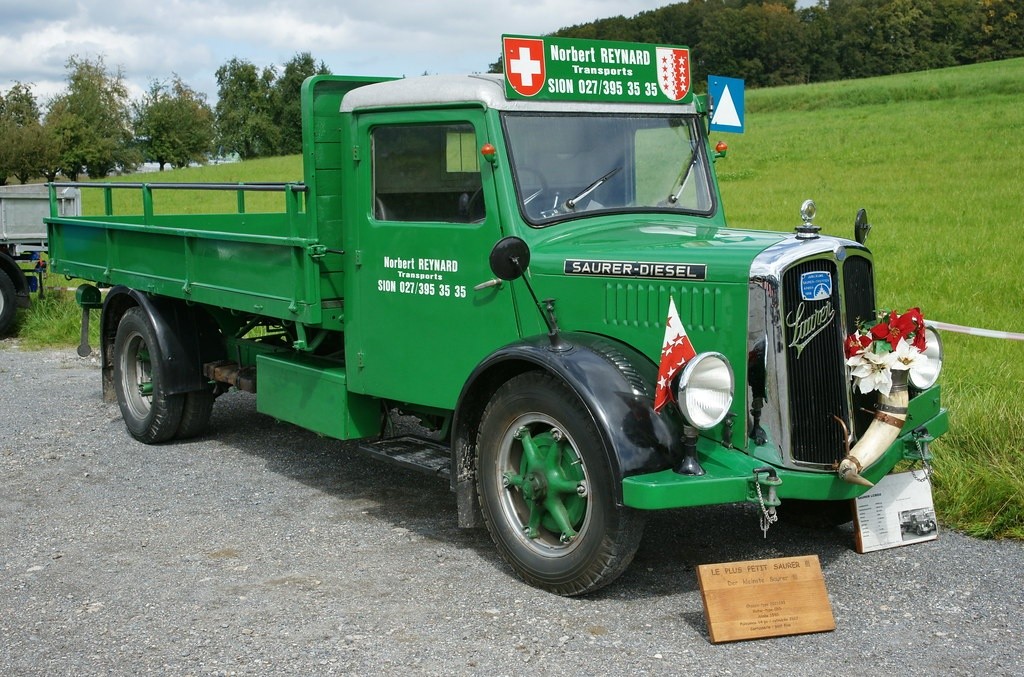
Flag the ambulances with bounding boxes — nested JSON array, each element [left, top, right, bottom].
[[44, 32, 949, 598]]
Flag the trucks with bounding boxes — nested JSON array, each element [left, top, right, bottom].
[[0, 181, 83, 339]]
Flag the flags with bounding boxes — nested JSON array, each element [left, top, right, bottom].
[[653, 299, 695, 413]]
